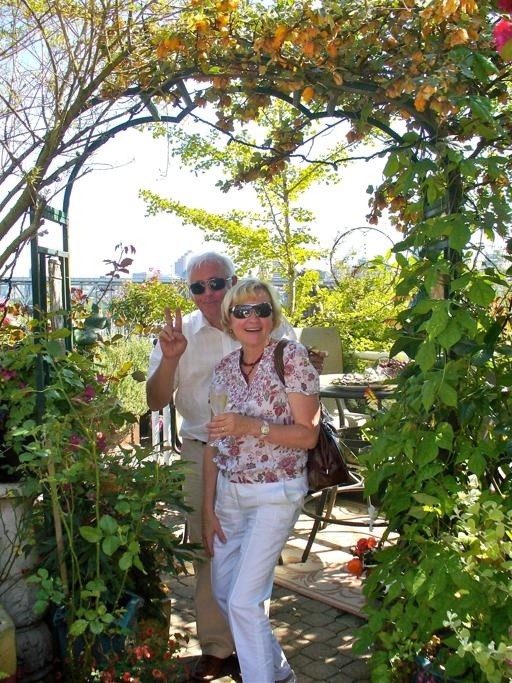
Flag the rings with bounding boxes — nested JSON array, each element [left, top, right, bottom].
[[221, 425, 225, 432]]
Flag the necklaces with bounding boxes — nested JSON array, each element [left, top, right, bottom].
[[239, 348, 263, 366], [239, 359, 254, 381]]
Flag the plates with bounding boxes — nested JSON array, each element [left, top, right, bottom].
[[332, 379, 388, 391]]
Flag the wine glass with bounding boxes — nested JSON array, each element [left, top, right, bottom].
[[207, 385, 230, 449]]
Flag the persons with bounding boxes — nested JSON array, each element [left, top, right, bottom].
[[144, 250, 329, 683], [201, 278, 324, 683]]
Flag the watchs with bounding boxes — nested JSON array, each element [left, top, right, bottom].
[[257, 417, 270, 440]]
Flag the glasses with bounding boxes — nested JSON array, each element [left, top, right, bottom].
[[229, 302, 274, 318], [188, 277, 232, 293]]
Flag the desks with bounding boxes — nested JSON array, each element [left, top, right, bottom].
[[300, 374, 402, 563]]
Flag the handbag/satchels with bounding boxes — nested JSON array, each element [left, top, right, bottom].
[[307, 416, 352, 495]]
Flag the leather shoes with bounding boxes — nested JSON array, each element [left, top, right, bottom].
[[191, 653, 224, 682]]
